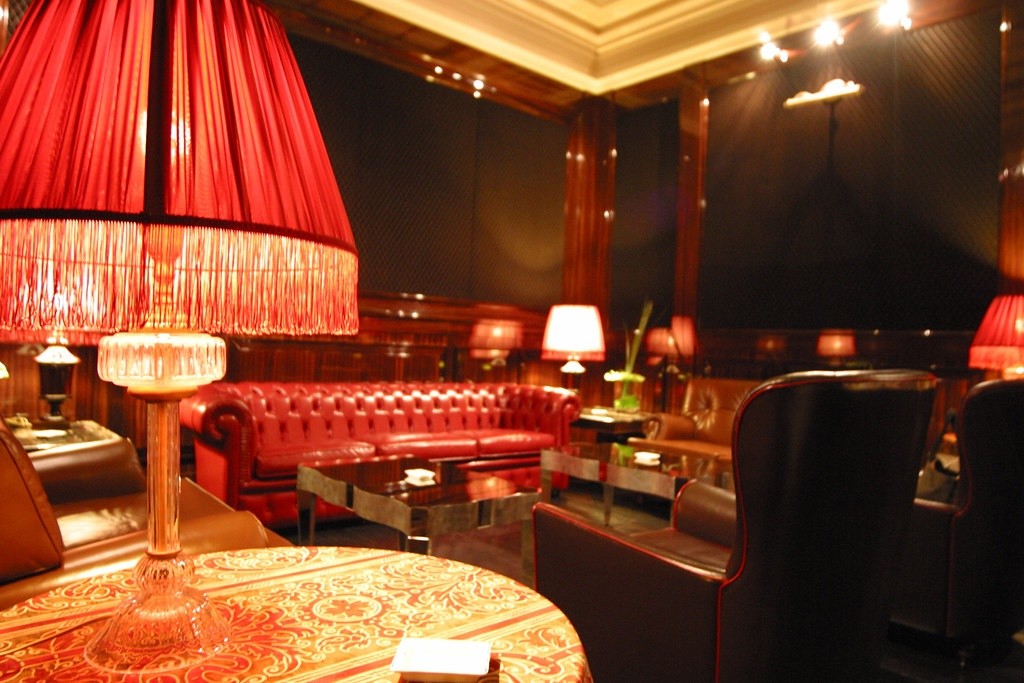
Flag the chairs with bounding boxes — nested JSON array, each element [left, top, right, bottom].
[[0, 421, 269, 610], [532, 369, 1024, 683]]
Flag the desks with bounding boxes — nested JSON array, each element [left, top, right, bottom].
[[297, 458, 543, 557], [4, 418, 121, 450], [0, 546, 593, 683]]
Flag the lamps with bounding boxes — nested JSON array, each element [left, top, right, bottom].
[[967, 294, 1024, 378], [0, 0, 360, 674], [540, 305, 605, 372], [33, 345, 80, 423]]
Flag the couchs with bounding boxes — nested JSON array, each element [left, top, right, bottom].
[[633, 379, 948, 492], [179, 382, 583, 527]]
